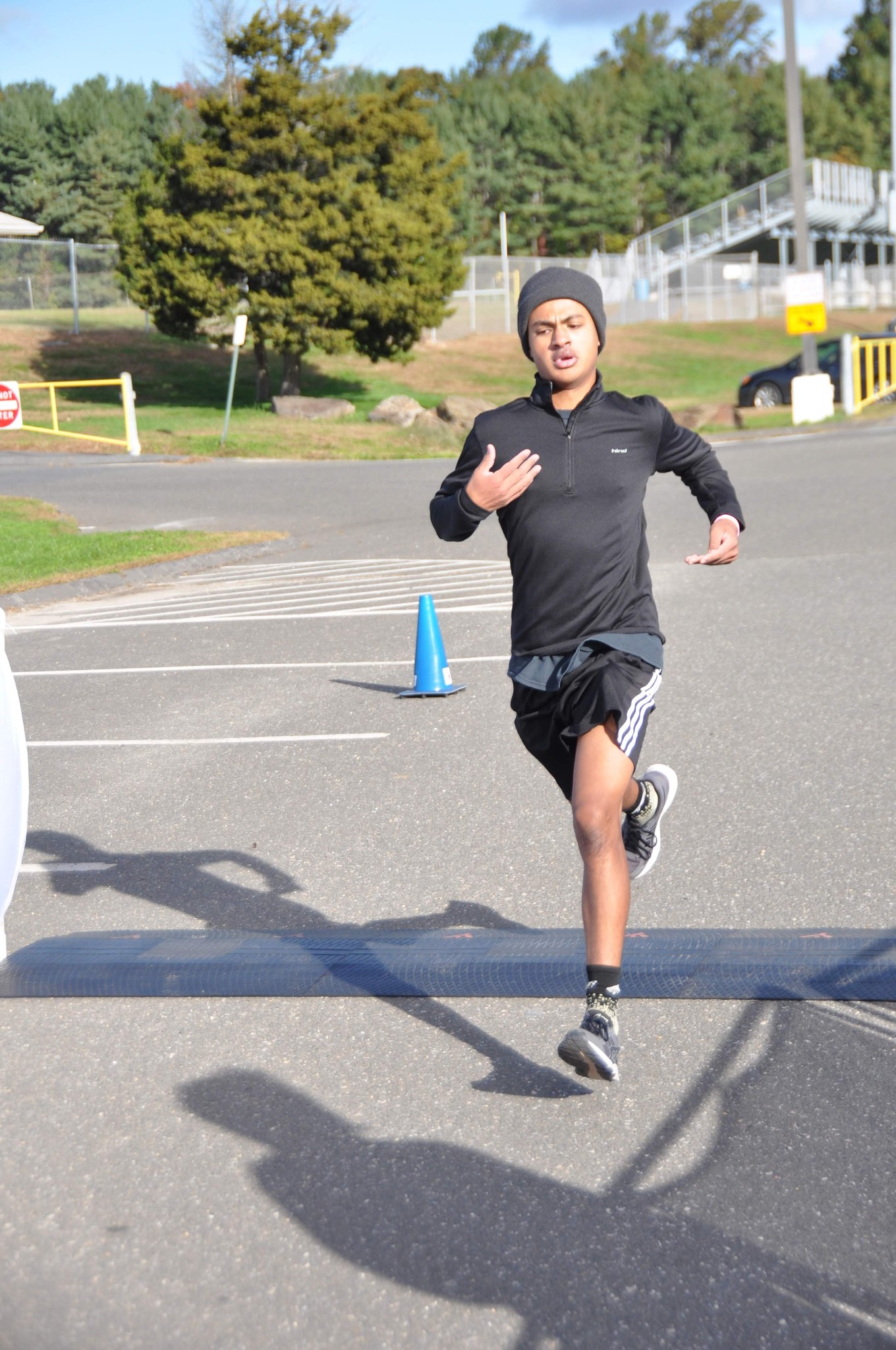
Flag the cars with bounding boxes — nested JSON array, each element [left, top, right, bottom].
[[738, 333, 895, 408]]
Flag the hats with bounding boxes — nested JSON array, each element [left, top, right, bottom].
[[518, 266, 606, 362]]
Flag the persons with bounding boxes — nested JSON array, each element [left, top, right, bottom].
[[427, 267, 744, 1083]]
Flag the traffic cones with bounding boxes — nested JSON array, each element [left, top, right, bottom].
[[395, 595, 465, 698]]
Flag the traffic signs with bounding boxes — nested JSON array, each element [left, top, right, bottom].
[[0, 372, 27, 432]]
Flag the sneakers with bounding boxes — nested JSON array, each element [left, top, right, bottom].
[[621, 763, 678, 879], [558, 1014, 620, 1081]]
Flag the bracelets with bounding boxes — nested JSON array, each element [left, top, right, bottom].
[[713, 514, 740, 537]]
